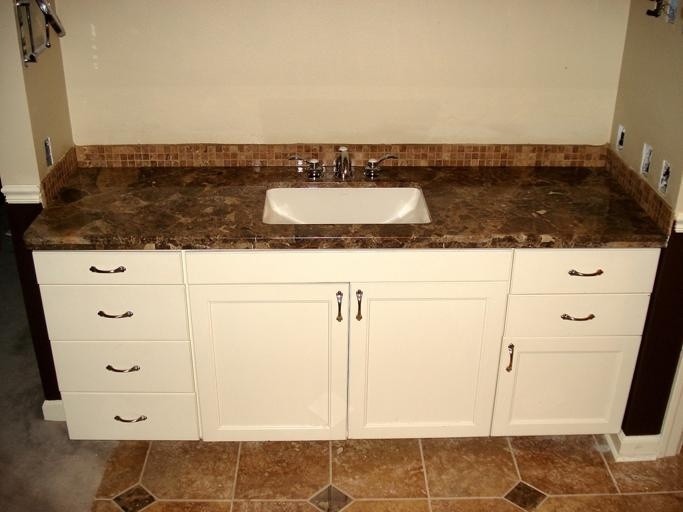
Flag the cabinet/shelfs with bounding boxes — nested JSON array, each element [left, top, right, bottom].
[[28, 250, 202, 439], [490, 249, 662, 440], [184, 250, 514, 440]]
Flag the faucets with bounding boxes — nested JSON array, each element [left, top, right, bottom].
[[336, 146, 353, 180]]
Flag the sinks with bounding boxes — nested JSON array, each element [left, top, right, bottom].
[[262, 181, 433, 225]]
